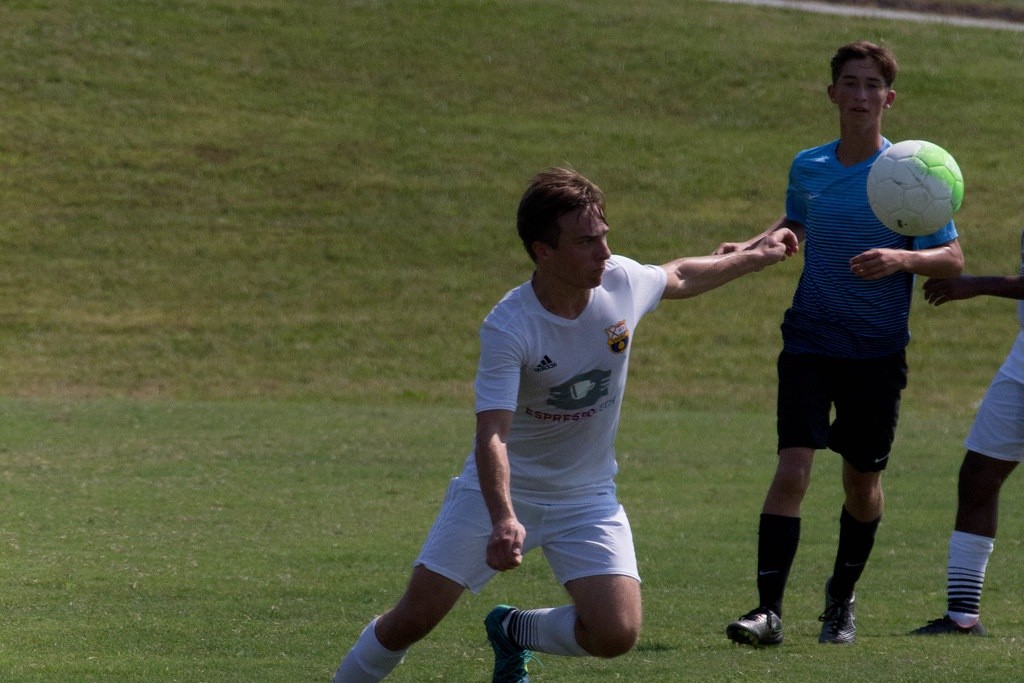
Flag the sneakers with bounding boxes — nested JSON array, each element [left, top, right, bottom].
[[483, 604, 545, 683], [817, 576, 855, 643], [909, 614, 985, 636], [726, 606, 784, 646]]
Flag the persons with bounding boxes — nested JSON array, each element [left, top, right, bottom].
[[713, 43, 965, 648], [910, 227, 1024, 637], [331, 167, 799, 683]]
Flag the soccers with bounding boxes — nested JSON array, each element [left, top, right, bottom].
[[865, 138, 968, 239]]
